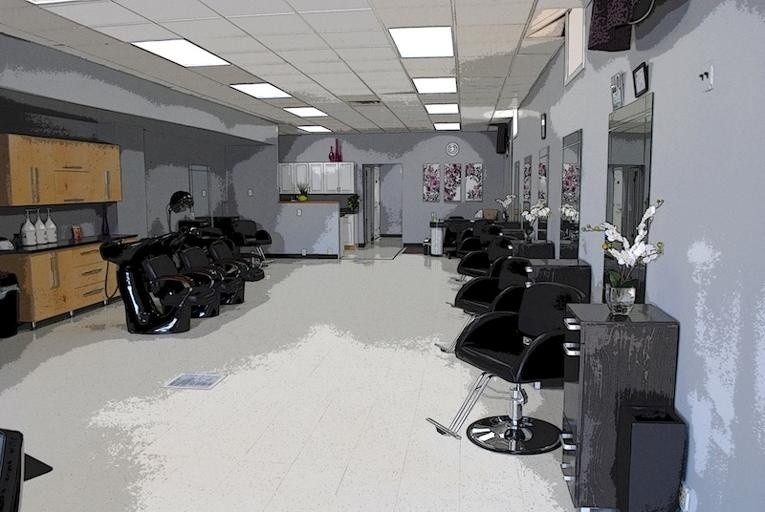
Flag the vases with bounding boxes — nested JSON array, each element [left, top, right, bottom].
[[605, 282, 636, 319]]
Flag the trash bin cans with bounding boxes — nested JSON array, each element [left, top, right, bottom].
[[0, 272, 20, 340], [423, 237, 431, 255]]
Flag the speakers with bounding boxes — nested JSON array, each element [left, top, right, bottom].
[[496, 122, 509, 154]]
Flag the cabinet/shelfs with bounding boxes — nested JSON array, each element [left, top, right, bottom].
[[278, 162, 355, 195], [0, 235, 139, 330], [559, 300, 681, 511], [0, 135, 122, 206]]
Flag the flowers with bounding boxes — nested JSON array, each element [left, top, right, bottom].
[[494, 193, 517, 215]]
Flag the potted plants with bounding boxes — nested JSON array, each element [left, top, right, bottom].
[[295, 181, 312, 202], [345, 194, 360, 212]]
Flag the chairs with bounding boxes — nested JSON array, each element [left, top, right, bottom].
[[99, 216, 272, 335], [425, 216, 591, 456]]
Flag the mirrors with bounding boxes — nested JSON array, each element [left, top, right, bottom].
[[512, 91, 655, 303]]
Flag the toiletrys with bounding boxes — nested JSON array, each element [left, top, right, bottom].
[[21, 208, 38, 247], [35, 207, 49, 245]]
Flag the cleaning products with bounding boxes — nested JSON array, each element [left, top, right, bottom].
[[45, 207, 58, 243]]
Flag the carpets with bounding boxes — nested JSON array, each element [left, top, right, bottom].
[[340, 237, 405, 260]]
